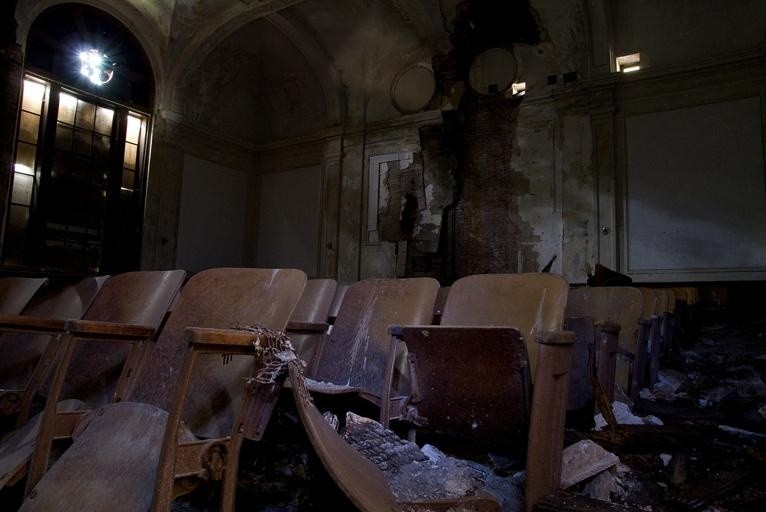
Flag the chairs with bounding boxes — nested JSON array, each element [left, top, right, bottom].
[[0, 267, 697, 512]]
